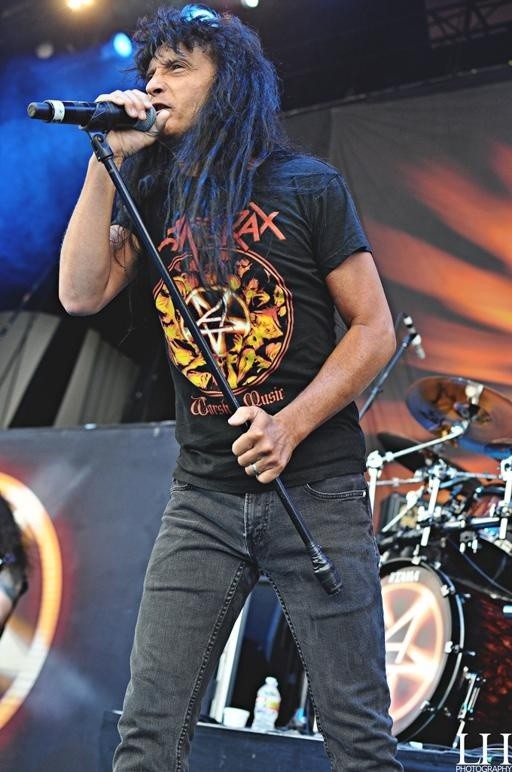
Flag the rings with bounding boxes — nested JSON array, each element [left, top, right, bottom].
[[253, 464, 258, 476]]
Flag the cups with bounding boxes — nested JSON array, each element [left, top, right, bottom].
[[223, 707, 250, 729]]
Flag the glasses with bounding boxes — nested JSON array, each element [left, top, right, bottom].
[[178, 3, 225, 29]]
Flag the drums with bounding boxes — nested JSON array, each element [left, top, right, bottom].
[[442, 527, 512, 597], [379, 558, 512, 750]]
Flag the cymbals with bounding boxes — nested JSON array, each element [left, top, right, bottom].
[[377, 430, 469, 492], [406, 374, 512, 451], [482, 439, 512, 461]]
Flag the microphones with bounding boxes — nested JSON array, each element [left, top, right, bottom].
[[28, 100, 156, 132], [403, 312, 426, 360]]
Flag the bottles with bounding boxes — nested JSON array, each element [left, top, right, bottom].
[[251, 676, 281, 734]]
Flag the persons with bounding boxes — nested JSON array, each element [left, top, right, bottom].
[[59, 6, 398, 772]]
